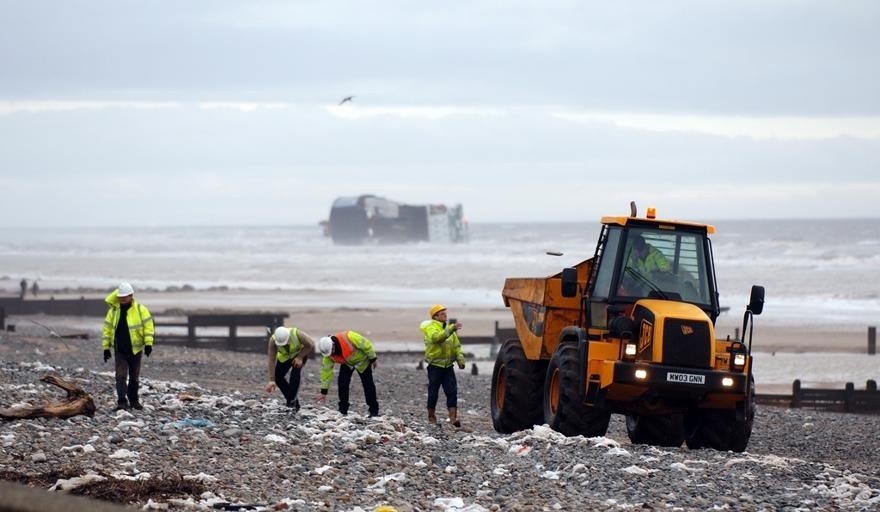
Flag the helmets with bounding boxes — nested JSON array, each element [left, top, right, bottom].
[[116, 282, 134, 298], [274, 326, 289, 346], [428, 304, 447, 319], [318, 337, 333, 357]]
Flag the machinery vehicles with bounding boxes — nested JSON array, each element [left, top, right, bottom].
[[482, 198, 766, 456]]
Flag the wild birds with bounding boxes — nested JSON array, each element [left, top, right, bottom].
[[340, 96, 353, 104]]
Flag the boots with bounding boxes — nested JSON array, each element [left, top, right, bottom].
[[447, 407, 461, 427], [427, 409, 441, 427]]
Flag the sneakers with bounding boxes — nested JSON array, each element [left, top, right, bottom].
[[366, 412, 378, 418], [336, 409, 348, 417], [112, 402, 143, 411]]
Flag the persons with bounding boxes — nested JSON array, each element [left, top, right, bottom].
[[316, 332, 379, 418], [103, 281, 156, 412], [419, 304, 465, 427], [264, 326, 314, 410], [624, 236, 674, 291]]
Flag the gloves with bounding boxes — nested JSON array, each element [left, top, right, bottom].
[[144, 345, 152, 357], [103, 349, 111, 362]]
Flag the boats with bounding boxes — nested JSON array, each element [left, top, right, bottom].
[[320, 190, 470, 245]]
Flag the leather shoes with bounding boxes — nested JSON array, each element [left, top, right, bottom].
[[285, 395, 301, 412]]
